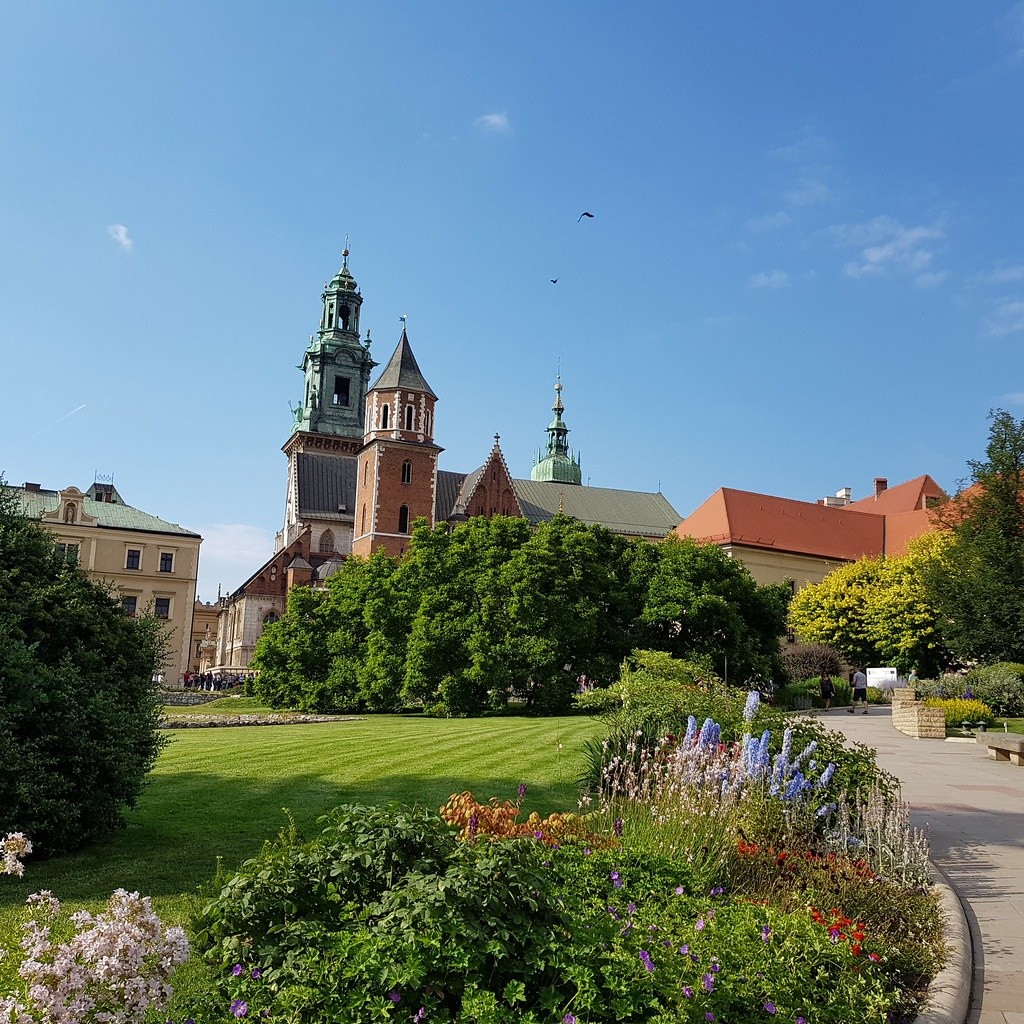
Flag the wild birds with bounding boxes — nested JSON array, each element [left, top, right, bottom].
[[577, 212, 594, 223], [550, 278, 560, 284]]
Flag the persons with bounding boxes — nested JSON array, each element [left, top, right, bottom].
[[183, 670, 259, 691], [151, 668, 167, 685], [820, 670, 836, 712], [846, 666, 869, 714]]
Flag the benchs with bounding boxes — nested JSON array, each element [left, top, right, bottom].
[[977, 732, 1024, 766]]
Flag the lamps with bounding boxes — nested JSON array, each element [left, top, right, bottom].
[[976, 721, 987, 732], [961, 721, 971, 730]]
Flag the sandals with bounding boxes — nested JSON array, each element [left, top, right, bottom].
[[862, 711, 868, 714], [848, 709, 854, 713]]
